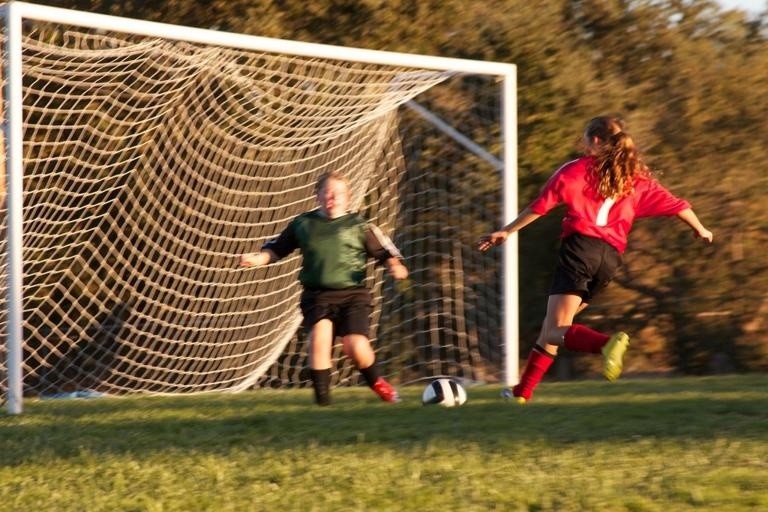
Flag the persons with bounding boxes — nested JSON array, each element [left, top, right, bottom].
[[239, 170, 408, 406], [475, 114, 714, 404]]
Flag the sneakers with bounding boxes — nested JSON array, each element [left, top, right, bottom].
[[601, 332, 630, 383], [368, 377, 401, 404], [500, 386, 529, 406]]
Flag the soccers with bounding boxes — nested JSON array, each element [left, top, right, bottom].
[[423, 378, 467, 407]]
